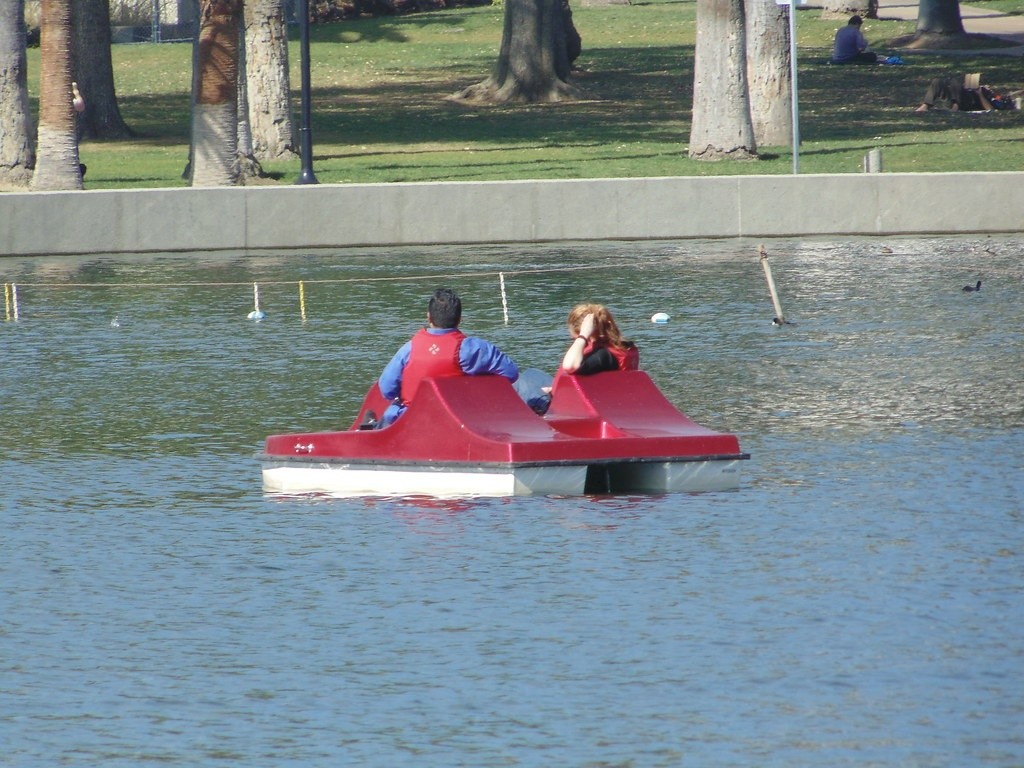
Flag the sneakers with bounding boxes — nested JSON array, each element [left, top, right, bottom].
[[360, 409, 377, 431]]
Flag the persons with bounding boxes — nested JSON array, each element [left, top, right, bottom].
[[918, 72, 1014, 117], [509, 302, 638, 416], [379, 288, 521, 434], [833, 16, 878, 64]]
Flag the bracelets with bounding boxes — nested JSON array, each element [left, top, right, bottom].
[[577, 334, 588, 348]]
